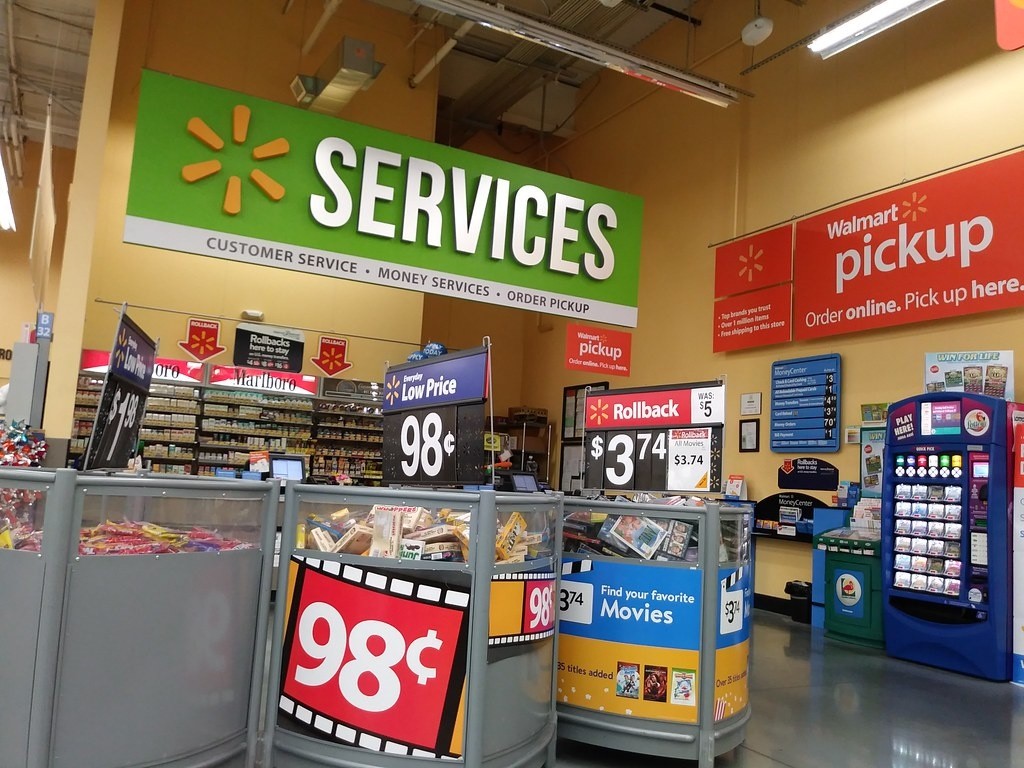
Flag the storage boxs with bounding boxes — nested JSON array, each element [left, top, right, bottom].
[[0, 464, 284, 768]]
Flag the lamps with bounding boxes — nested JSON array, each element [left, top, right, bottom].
[[408, 0, 766, 110], [798, 1, 950, 62]]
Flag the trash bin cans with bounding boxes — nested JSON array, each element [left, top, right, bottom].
[[784, 580, 812, 624]]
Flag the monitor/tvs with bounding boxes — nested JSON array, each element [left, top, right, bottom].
[[270, 454, 305, 484], [513, 472, 540, 492]]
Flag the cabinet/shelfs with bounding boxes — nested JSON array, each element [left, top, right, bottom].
[[198, 363, 320, 484], [537, 490, 759, 767], [260, 470, 561, 768], [65, 347, 206, 481], [487, 420, 553, 486], [314, 375, 386, 485]]
